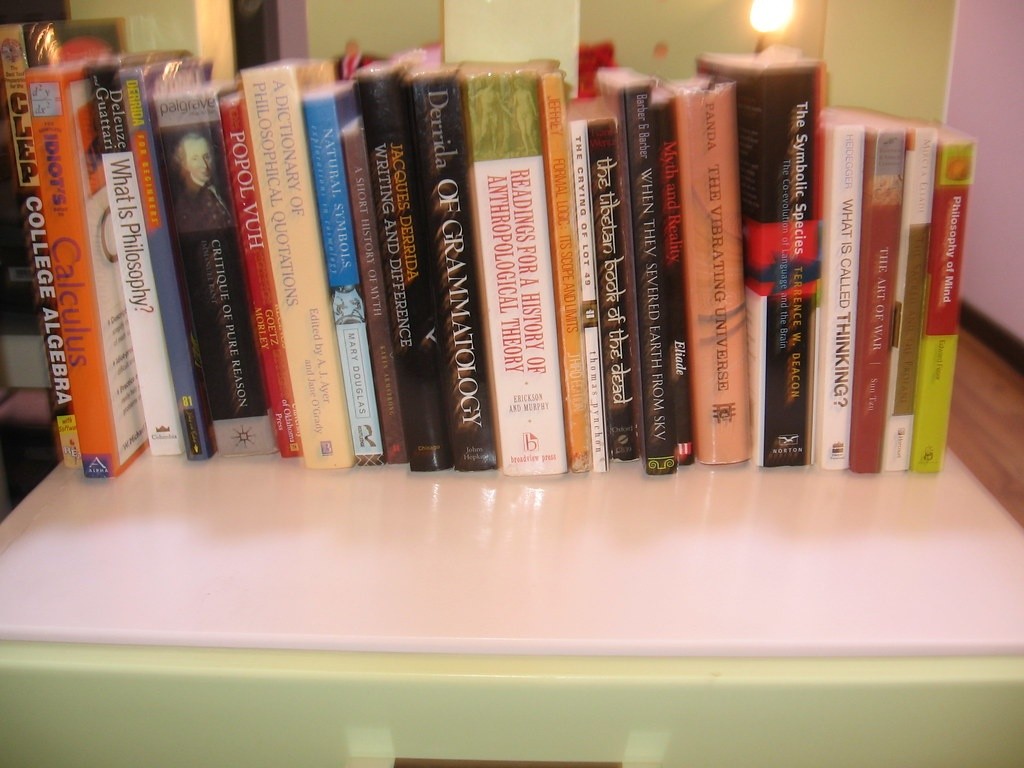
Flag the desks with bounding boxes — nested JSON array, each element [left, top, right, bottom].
[[0, 331, 1023, 768]]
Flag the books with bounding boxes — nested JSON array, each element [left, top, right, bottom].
[[910, 119, 976, 471], [241, 56, 355, 470], [1, 17, 690, 475], [462, 61, 567, 475], [668, 75, 751, 465], [817, 106, 933, 473], [693, 52, 828, 468]]
